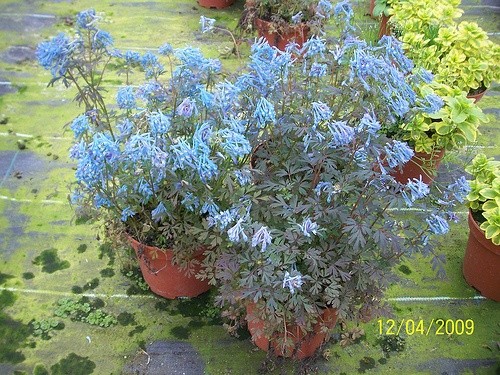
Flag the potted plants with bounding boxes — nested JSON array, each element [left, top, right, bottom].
[[371, 73, 491, 200], [436, 21, 500, 102], [459, 152, 500, 304], [245, 0, 325, 59]]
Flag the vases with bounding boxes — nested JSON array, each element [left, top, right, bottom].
[[121, 193, 218, 298], [241, 272, 342, 360], [197, 0, 238, 11]]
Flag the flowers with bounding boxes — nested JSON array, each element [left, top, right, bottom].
[[39, 14, 470, 296]]
